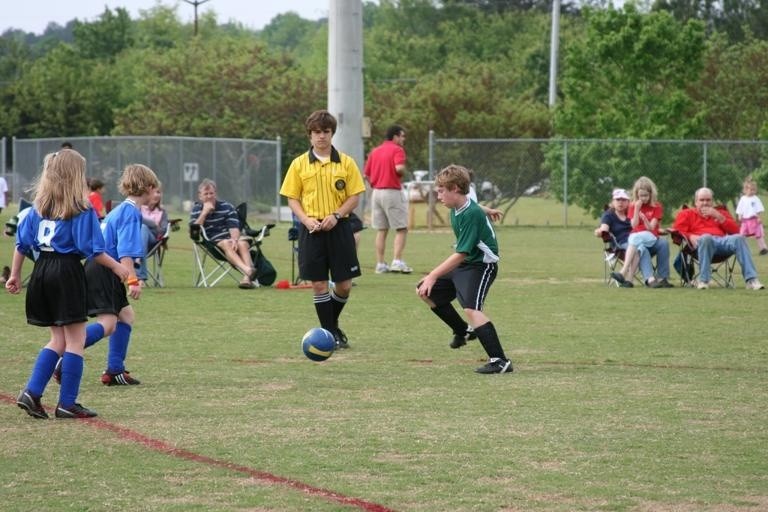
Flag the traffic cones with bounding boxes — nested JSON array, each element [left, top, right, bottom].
[[0, 264, 10, 287]]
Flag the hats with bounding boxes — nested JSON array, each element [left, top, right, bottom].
[[611, 189, 629, 201]]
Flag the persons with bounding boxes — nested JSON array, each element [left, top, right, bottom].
[[593, 187, 674, 289], [86, 179, 106, 221], [133, 182, 167, 289], [275, 111, 368, 350], [0, 177, 8, 213], [608, 177, 664, 287], [736, 181, 768, 257], [6, 149, 128, 420], [188, 177, 257, 288], [361, 125, 414, 276], [51, 162, 159, 387], [673, 187, 766, 291], [414, 165, 517, 375]]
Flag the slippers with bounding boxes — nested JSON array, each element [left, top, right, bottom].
[[237, 267, 260, 290]]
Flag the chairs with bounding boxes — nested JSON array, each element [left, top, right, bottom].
[[282, 204, 373, 287], [595, 199, 672, 291], [658, 200, 742, 292], [100, 196, 186, 289], [16, 196, 37, 291], [187, 201, 283, 289]]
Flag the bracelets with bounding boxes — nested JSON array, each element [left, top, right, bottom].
[[332, 213, 341, 221]]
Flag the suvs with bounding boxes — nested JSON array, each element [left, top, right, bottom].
[[408, 163, 499, 205], [3, 173, 29, 202]]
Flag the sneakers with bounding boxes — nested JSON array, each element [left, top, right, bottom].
[[474, 359, 514, 376], [53, 356, 64, 385], [648, 277, 676, 289], [17, 389, 49, 420], [55, 403, 98, 420], [102, 368, 141, 388], [449, 325, 477, 349], [376, 259, 414, 275], [697, 278, 709, 290], [743, 277, 765, 292], [610, 272, 634, 287], [327, 327, 351, 350]]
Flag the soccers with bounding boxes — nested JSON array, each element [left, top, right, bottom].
[[302, 328, 335, 363]]
